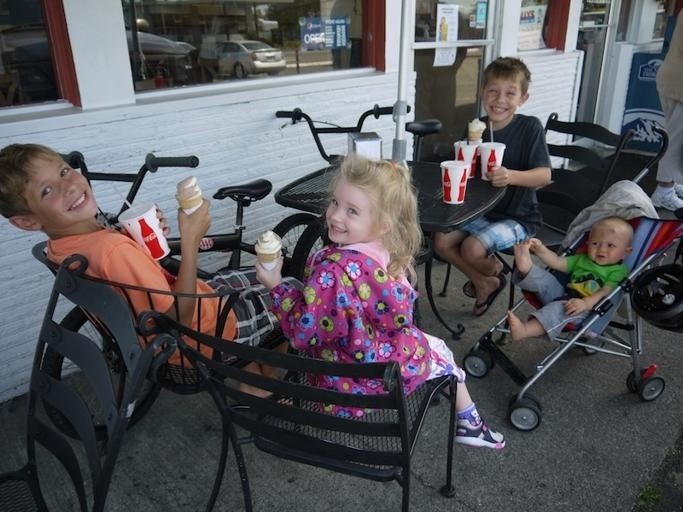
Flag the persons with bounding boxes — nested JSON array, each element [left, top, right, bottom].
[[256, 155, 505, 450], [507, 215, 634, 342], [434, 57, 553, 316], [1, 145, 304, 403], [651, 7, 683, 211]]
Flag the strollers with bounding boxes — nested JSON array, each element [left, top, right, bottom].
[[458, 124, 683, 434]]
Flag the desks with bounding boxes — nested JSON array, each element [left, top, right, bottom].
[[273, 164, 508, 338]]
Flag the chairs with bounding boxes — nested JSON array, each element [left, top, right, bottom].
[[132, 308, 457, 512], [1, 255, 178, 512], [440, 113, 634, 346], [31, 239, 292, 510]]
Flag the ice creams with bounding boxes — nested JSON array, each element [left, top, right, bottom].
[[174, 175, 203, 216], [255, 229, 282, 271], [468, 118, 487, 142]]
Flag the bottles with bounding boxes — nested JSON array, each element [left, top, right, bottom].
[[469, 147, 476, 176], [456, 168, 467, 201], [443, 167, 452, 202], [137, 218, 165, 258], [456, 147, 464, 161], [486, 147, 496, 173]]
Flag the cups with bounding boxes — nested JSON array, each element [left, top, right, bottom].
[[439, 160, 471, 204], [116, 202, 171, 261], [453, 141, 477, 179], [478, 142, 507, 182]]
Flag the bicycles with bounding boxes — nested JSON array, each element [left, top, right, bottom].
[[42, 144, 328, 440], [273, 105, 450, 299]]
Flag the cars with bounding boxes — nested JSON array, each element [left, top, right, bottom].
[[123, 29, 213, 100], [198, 39, 288, 79]]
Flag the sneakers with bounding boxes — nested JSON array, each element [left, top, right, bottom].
[[454, 419, 507, 449], [673, 183, 683, 197], [651, 189, 683, 212]]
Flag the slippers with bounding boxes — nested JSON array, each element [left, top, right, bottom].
[[463, 274, 506, 317], [229, 393, 294, 415]]
